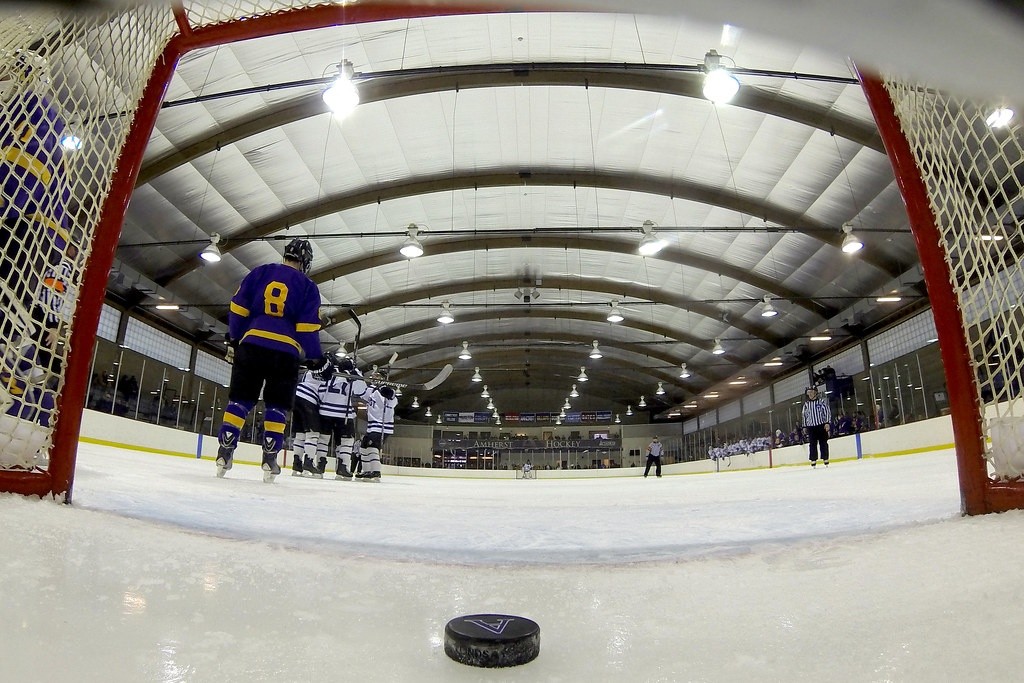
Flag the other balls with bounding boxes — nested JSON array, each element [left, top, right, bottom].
[[443, 614, 541, 668]]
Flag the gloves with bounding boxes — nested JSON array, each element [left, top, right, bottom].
[[224, 346, 236, 365], [310, 357, 337, 381], [378, 387, 397, 400]]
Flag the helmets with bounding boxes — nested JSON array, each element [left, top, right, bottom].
[[283, 238, 313, 274], [338, 358, 356, 377], [370, 368, 387, 386], [4, 48, 51, 92], [805, 385, 818, 395]]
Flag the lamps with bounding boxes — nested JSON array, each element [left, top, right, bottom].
[[607, 301, 625, 322], [702, 49, 740, 102], [400, 223, 430, 257], [762, 295, 778, 317], [412, 337, 782, 424], [200, 232, 228, 262], [62, 125, 85, 149], [983, 106, 1014, 128], [639, 220, 659, 253], [436, 299, 455, 323], [322, 59, 359, 111], [841, 221, 864, 253]]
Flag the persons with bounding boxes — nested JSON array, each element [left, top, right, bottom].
[[534, 435, 573, 441], [380, 456, 431, 468], [0, 48, 81, 467], [709, 422, 809, 461], [534, 462, 635, 470], [150, 396, 177, 425], [828, 404, 915, 438], [494, 463, 525, 470], [216, 239, 333, 482], [524, 460, 532, 479], [89, 370, 139, 416], [463, 433, 529, 440], [802, 385, 832, 469], [644, 437, 664, 478], [291, 352, 399, 482]]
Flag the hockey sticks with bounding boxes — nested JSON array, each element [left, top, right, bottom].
[[330, 363, 454, 390], [345, 309, 361, 425], [379, 352, 398, 458]]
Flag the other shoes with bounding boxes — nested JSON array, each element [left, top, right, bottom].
[[643, 476, 647, 478], [656, 474, 662, 478]]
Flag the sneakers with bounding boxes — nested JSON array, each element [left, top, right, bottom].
[[335, 458, 353, 480], [355, 471, 374, 483], [291, 454, 303, 476], [372, 471, 381, 483], [302, 454, 323, 478], [261, 438, 281, 484], [811, 461, 817, 469], [823, 460, 829, 468], [316, 457, 326, 473], [216, 432, 235, 478]]
